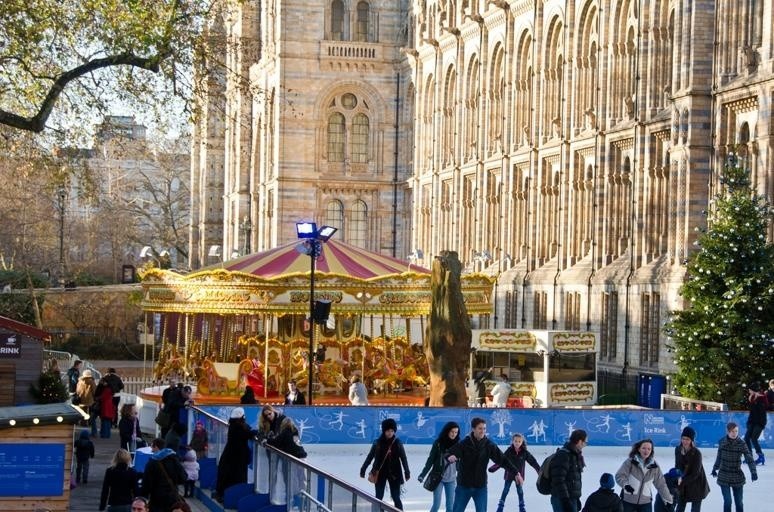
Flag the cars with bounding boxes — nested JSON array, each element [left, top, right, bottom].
[[41, 266, 77, 289]]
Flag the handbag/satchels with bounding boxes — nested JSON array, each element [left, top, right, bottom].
[[71, 393, 80, 404], [368, 470, 380, 483], [424, 470, 443, 492], [155, 409, 170, 428]]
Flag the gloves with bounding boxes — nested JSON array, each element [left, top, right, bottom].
[[751, 473, 757, 482], [624, 484, 634, 494], [711, 470, 717, 477]]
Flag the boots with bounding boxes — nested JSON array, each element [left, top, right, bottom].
[[519, 500, 526, 512], [496, 500, 504, 512], [755, 453, 765, 463]]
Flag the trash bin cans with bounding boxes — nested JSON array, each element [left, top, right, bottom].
[[122, 265, 134, 283], [637, 373, 666, 410]]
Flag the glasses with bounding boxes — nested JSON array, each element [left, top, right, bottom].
[[133, 495, 149, 504], [264, 412, 272, 416]]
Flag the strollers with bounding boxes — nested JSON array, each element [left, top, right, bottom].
[[126, 437, 155, 490], [90, 391, 122, 429]]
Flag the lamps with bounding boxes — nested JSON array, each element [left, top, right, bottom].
[[207, 243, 227, 270], [406, 245, 423, 272], [140, 243, 162, 272]]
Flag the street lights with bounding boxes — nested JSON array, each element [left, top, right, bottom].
[[140, 246, 170, 381], [54, 184, 73, 292], [208, 245, 241, 262], [296, 221, 339, 405]]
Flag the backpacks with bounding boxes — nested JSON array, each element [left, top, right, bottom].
[[537, 447, 572, 495]]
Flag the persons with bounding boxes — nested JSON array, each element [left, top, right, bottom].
[[239, 385, 259, 404], [442, 418, 524, 512], [491, 374, 513, 407], [418, 422, 460, 512], [467, 365, 495, 408], [284, 379, 307, 405], [347, 375, 368, 406], [359, 418, 411, 512], [490, 434, 540, 511], [551, 383, 774, 512], [213, 405, 251, 505], [256, 405, 299, 502], [44, 359, 209, 511]]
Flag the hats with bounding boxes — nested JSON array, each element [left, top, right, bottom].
[[749, 382, 760, 392], [682, 426, 696, 442], [382, 419, 397, 433], [669, 468, 683, 478], [230, 407, 245, 419], [78, 370, 94, 379], [600, 473, 615, 488]]
